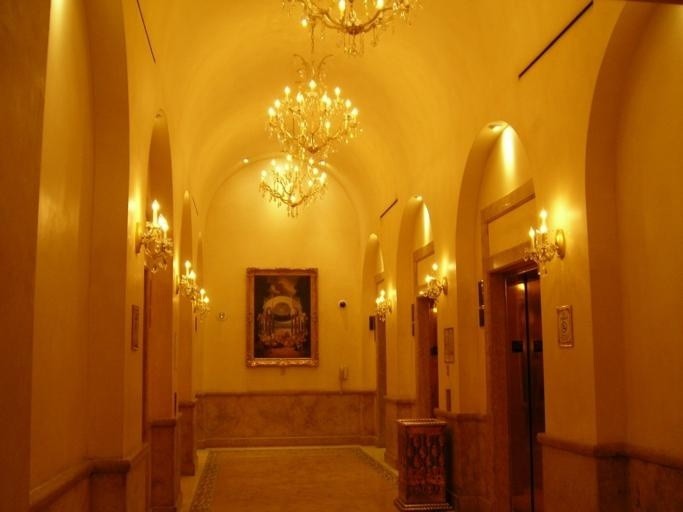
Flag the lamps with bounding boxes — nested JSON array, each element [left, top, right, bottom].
[[376, 290, 392, 323], [423, 263, 448, 309], [176, 260, 210, 322], [519, 207, 565, 276], [258, 0, 424, 218], [134, 199, 173, 275]]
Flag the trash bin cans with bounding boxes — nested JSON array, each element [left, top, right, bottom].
[[393, 418, 455, 512]]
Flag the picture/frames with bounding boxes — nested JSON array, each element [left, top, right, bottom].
[[443, 327, 455, 364], [556, 304, 574, 348], [130, 304, 140, 352], [244, 266, 321, 369]]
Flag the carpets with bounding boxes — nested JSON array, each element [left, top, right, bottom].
[[187, 447, 401, 512]]
